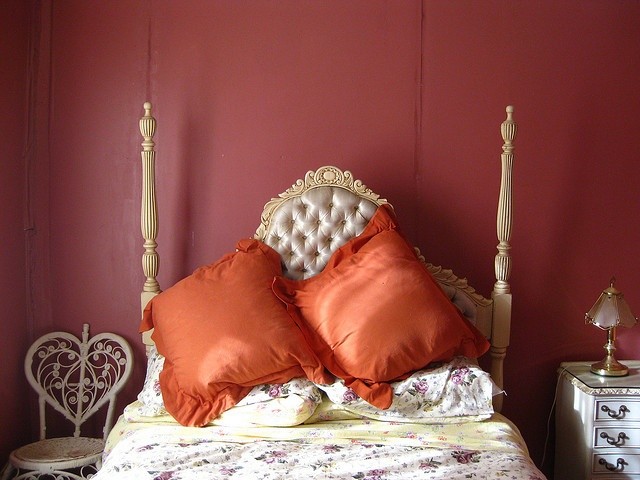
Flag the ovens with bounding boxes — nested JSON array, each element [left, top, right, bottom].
[[94, 102, 543, 479]]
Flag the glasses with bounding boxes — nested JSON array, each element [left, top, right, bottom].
[[584, 276, 638, 378]]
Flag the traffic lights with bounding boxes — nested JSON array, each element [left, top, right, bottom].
[[271, 203, 492, 409], [139, 238, 334, 427], [124, 346, 325, 428], [312, 357, 507, 426]]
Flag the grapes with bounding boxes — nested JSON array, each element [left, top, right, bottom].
[[553, 359, 640, 479]]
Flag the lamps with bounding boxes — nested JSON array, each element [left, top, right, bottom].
[[1, 323, 135, 480]]
